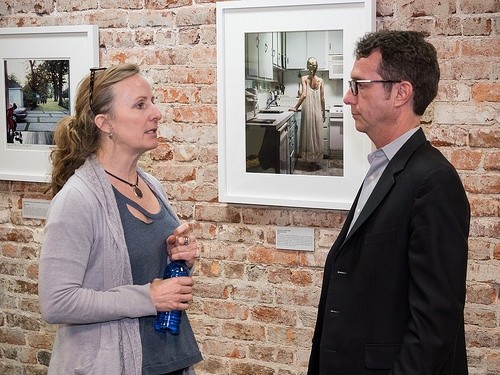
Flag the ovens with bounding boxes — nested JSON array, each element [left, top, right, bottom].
[[329, 117, 343, 150]]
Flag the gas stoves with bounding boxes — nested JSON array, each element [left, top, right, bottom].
[[329, 104, 343, 117]]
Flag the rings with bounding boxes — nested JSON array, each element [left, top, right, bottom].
[[184, 236, 189, 245]]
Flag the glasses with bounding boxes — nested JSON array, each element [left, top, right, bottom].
[[349, 79, 415, 96], [89, 67, 108, 116]]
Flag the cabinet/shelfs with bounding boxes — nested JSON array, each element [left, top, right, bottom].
[[246, 111, 329, 174], [245, 30, 343, 81]]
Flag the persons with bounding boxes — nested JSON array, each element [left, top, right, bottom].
[[38, 63, 198, 375], [306, 29, 471, 375]]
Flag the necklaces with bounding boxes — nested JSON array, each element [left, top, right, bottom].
[[104, 169, 143, 198]]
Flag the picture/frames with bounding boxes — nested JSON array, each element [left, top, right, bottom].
[[216, 0, 376, 211], [0, 25, 99, 182]]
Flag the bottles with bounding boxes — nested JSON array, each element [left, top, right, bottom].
[[153, 259, 190, 336]]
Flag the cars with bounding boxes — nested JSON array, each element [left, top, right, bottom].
[[13, 108, 28, 124]]
